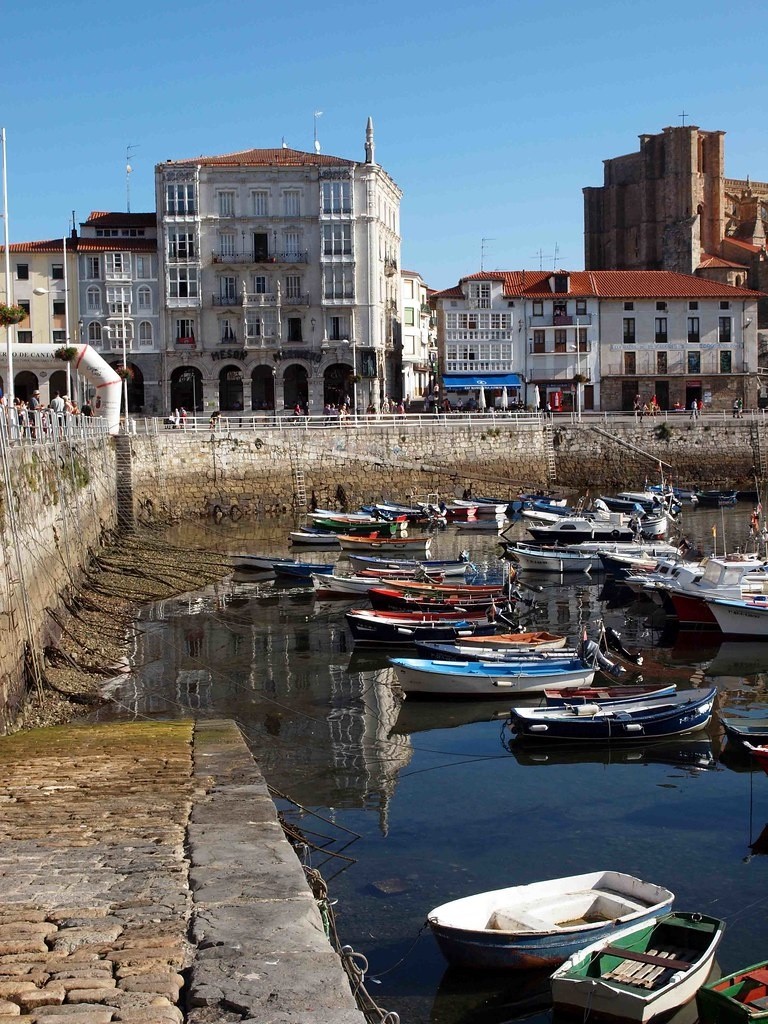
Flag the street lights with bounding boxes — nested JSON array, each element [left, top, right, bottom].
[[33, 287, 582, 423]]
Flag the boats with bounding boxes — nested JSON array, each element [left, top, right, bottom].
[[310, 554, 543, 645], [505, 683, 768, 775], [452, 520, 503, 529], [413, 627, 643, 666], [337, 549, 432, 562], [427, 870, 768, 1024], [429, 964, 554, 1024], [596, 502, 768, 639], [597, 579, 768, 678], [386, 640, 627, 700], [387, 695, 546, 739], [229, 554, 335, 577], [230, 572, 314, 589], [453, 528, 505, 536], [456, 630, 567, 650], [335, 534, 433, 550], [289, 497, 509, 544], [498, 483, 740, 572]]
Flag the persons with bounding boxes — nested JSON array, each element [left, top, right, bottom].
[[455, 397, 463, 410], [424, 394, 433, 409], [304, 402, 310, 422], [433, 382, 439, 397], [367, 394, 410, 425], [0, 389, 95, 438], [545, 401, 552, 418], [467, 398, 477, 409], [443, 398, 450, 411], [511, 398, 518, 404], [169, 407, 188, 429], [732, 397, 743, 418], [209, 411, 222, 429], [690, 398, 703, 419], [633, 394, 660, 416], [672, 402, 685, 409], [323, 394, 350, 426], [293, 404, 300, 426]]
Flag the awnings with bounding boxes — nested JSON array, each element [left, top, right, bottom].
[[441, 375, 522, 391]]
